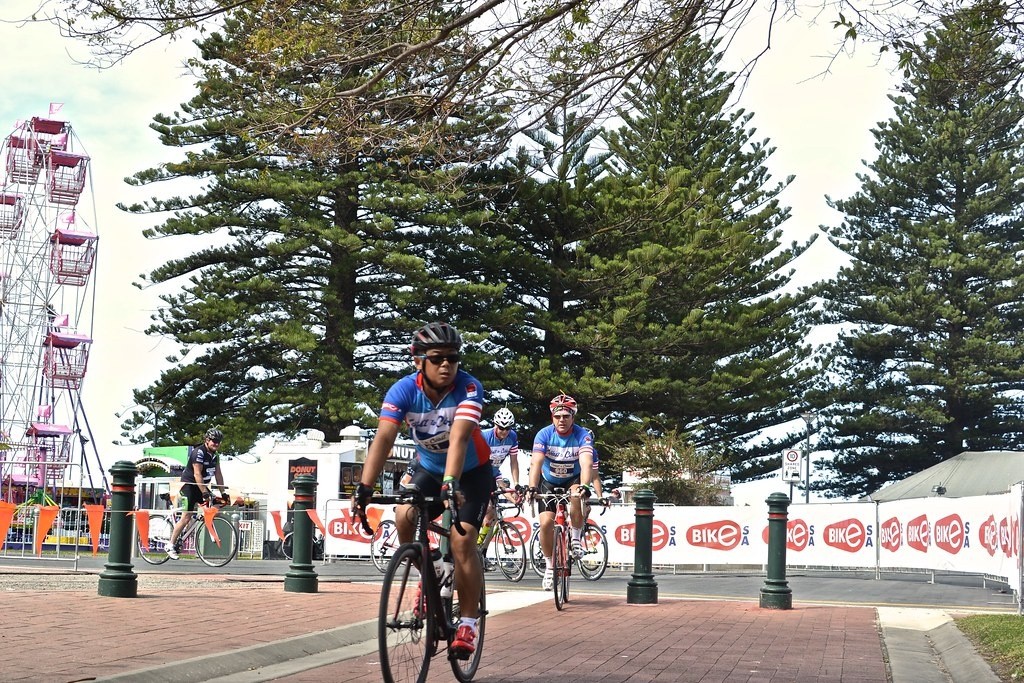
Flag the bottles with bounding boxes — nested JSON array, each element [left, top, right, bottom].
[[430, 546, 445, 584], [439, 553, 455, 599], [175, 518, 186, 529], [476, 522, 490, 544], [559, 527, 568, 549], [184, 515, 197, 531]]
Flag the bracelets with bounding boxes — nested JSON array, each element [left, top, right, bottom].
[[443, 476, 457, 481], [584, 484, 589, 488], [515, 482, 519, 484]]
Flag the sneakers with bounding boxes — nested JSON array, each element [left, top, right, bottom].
[[450, 625, 476, 653], [413, 580, 427, 618], [542, 567, 558, 591], [571, 536, 584, 560], [484, 558, 496, 572]]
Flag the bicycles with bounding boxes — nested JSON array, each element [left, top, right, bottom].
[[369, 489, 610, 582], [137, 493, 239, 567], [527, 491, 587, 611], [282, 518, 325, 560], [350, 489, 490, 682]]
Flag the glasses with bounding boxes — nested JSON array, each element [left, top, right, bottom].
[[419, 355, 459, 365], [209, 438, 220, 443], [552, 415, 571, 419], [499, 426, 511, 430]]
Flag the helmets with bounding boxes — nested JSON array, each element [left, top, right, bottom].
[[549, 394, 578, 414], [583, 427, 595, 440], [494, 408, 515, 428], [205, 428, 223, 439], [410, 322, 462, 355]]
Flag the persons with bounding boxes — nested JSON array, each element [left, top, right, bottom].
[[482, 409, 522, 571], [351, 323, 492, 655], [565, 427, 610, 545], [526, 395, 593, 591], [163, 428, 230, 559]]
